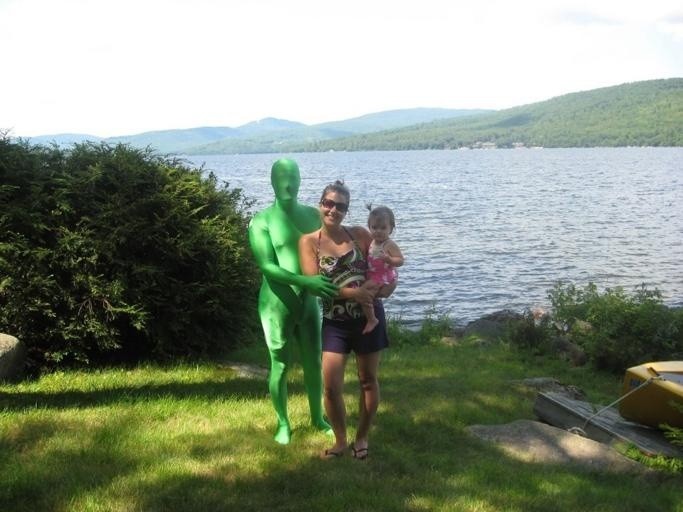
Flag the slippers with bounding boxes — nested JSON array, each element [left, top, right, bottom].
[[319, 444, 347, 461], [351, 442, 369, 459]]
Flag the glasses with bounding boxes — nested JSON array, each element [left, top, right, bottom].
[[322, 199, 349, 212]]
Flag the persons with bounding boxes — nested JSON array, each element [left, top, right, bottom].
[[297, 177, 390, 464], [356, 202, 403, 335], [247, 156, 340, 447]]
